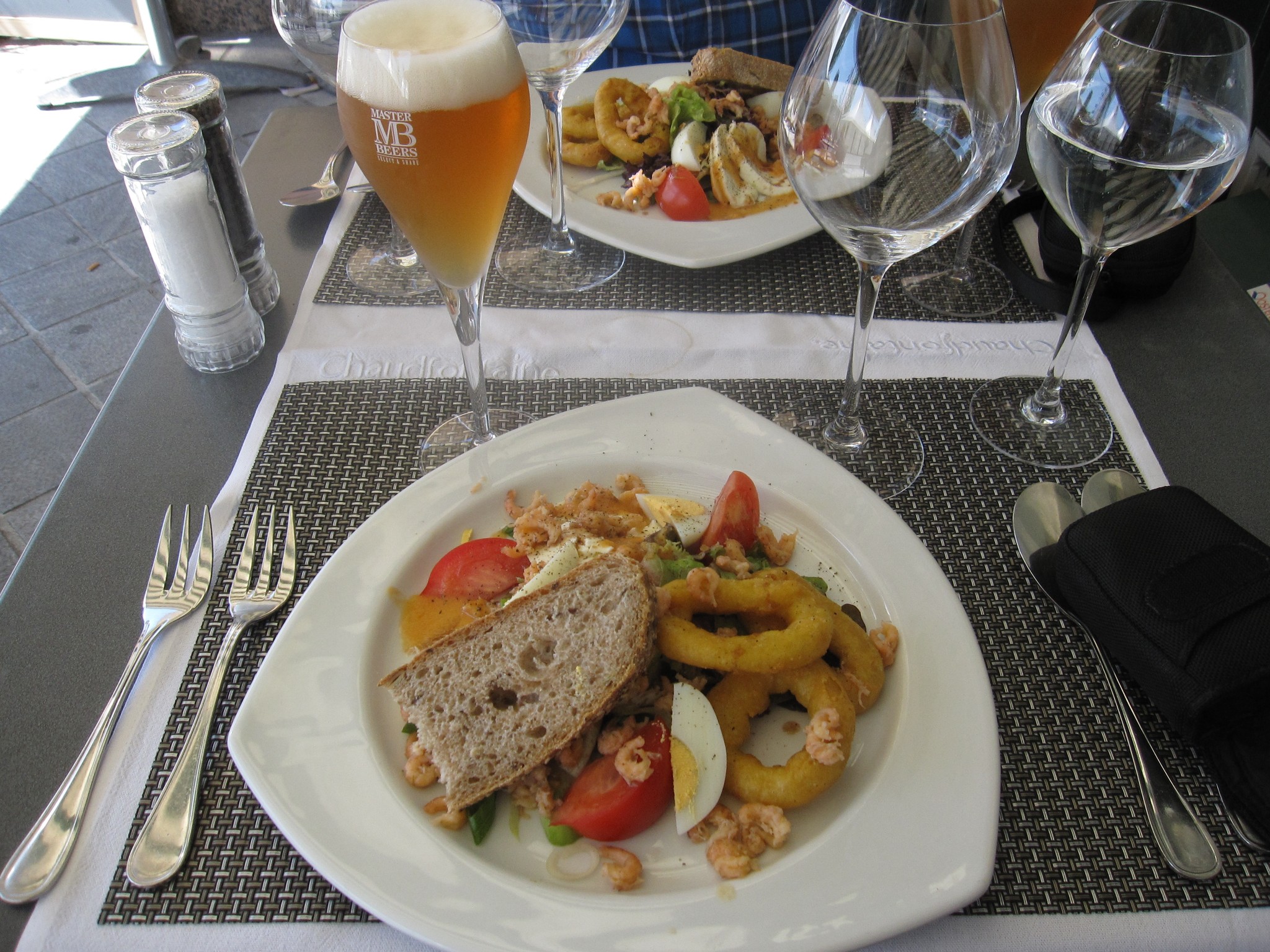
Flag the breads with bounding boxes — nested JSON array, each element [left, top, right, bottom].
[[689, 48, 794, 91], [377, 549, 662, 810]]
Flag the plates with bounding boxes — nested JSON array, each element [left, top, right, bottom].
[[226, 386, 1000, 952], [510, 59, 887, 272]]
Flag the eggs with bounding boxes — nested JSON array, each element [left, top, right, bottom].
[[506, 541, 579, 605], [650, 76, 799, 203], [636, 494, 711, 548], [672, 681, 728, 834]]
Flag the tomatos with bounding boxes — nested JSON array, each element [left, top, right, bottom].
[[421, 538, 532, 597], [554, 720, 670, 842], [701, 470, 760, 554], [658, 165, 710, 222]]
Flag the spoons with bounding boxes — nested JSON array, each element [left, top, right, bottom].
[[278, 125, 352, 209], [1013, 468, 1270, 878]]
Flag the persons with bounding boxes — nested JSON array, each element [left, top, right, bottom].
[[495, 0, 928, 85]]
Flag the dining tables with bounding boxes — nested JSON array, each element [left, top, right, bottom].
[[0, 103, 1270, 949]]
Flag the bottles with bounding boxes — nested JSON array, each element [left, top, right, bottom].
[[105, 113, 270, 376], [134, 71, 280, 327]]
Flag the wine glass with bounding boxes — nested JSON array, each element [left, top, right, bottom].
[[335, 0, 555, 484], [763, 2, 1022, 507], [899, 0, 1095, 318], [266, 0, 464, 297], [972, 0, 1253, 468], [482, 0, 634, 297]]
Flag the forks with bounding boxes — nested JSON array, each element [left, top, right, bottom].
[[0, 502, 214, 905], [126, 502, 305, 890]]
